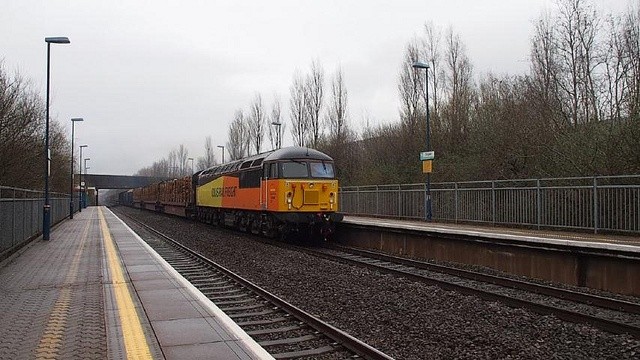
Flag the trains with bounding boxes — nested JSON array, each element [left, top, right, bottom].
[[109, 147, 344, 244]]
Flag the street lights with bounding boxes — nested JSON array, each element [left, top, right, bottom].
[[272, 122, 282, 148], [68, 118, 85, 220], [217, 145, 225, 164], [79, 145, 88, 213], [412, 62, 433, 222], [84, 158, 91, 209], [42, 36, 70, 242], [188, 157, 194, 175]]
